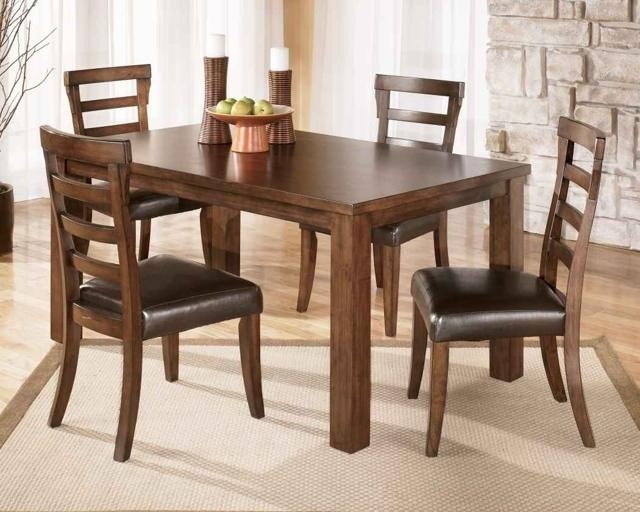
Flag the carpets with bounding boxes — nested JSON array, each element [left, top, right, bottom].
[[0, 338, 640, 512]]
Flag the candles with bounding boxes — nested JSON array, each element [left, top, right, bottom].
[[207, 32, 225, 58], [271, 46, 290, 72]]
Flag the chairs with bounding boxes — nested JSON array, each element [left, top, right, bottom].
[[297, 72, 466, 337], [405, 115, 608, 459], [40, 124, 264, 461], [63, 64, 214, 267]]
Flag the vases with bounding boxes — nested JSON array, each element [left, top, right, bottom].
[[0, 182, 13, 254]]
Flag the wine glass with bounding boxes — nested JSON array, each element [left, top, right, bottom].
[[206, 103, 293, 153]]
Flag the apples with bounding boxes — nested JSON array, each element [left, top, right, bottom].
[[252, 99, 272, 115], [239, 96, 253, 104], [231, 102, 251, 115], [216, 100, 233, 114], [226, 98, 236, 103]]
